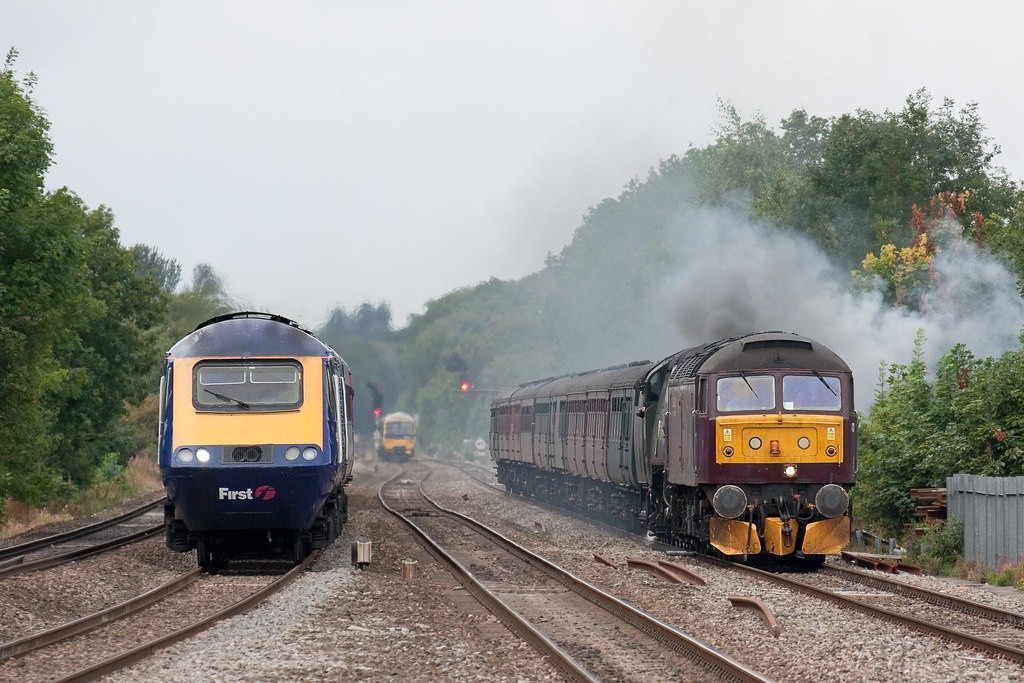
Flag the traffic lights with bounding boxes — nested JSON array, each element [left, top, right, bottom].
[[458, 377, 473, 394], [374, 398, 383, 417]]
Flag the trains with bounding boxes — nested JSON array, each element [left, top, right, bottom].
[[485, 330, 862, 565], [380, 412, 419, 460], [152, 312, 367, 574]]
